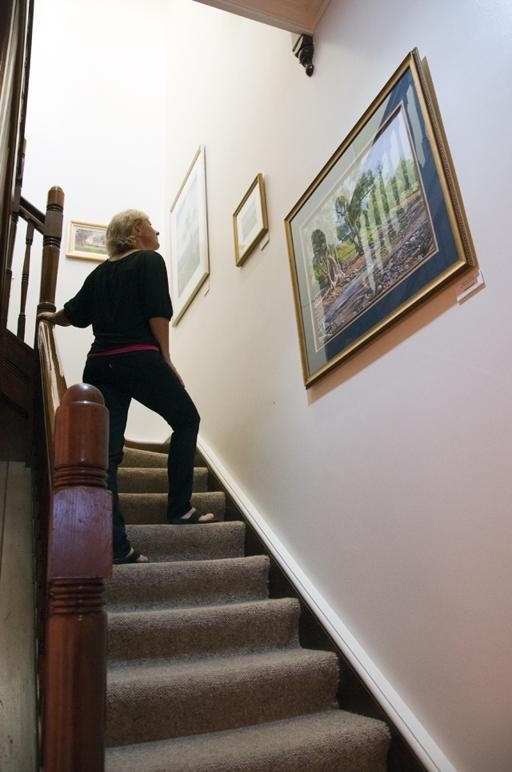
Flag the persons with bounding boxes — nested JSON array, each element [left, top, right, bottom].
[[37, 209, 215, 565]]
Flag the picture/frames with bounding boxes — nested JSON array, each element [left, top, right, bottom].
[[231, 171, 268, 268], [285, 47, 478, 389], [66, 219, 110, 261], [167, 142, 210, 327]]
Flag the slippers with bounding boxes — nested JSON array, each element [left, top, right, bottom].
[[167, 509, 219, 524], [112, 550, 149, 563]]
